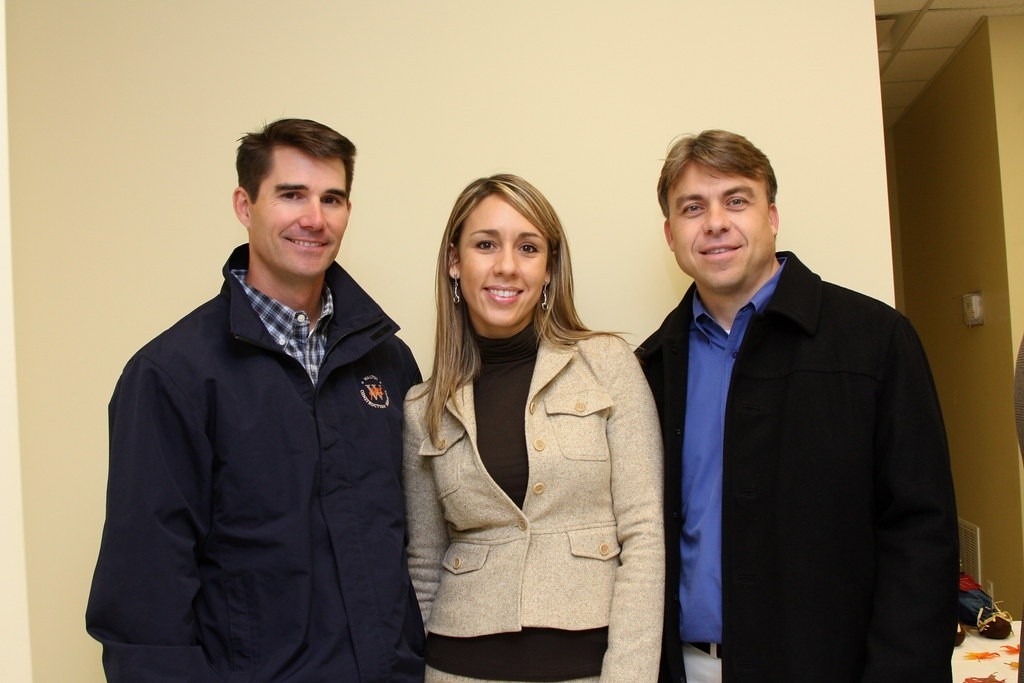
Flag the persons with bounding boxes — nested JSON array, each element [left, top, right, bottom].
[[402, 175, 665, 683], [632, 129, 960, 682], [85, 119, 424, 683]]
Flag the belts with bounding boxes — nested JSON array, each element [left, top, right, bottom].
[[690, 642, 723, 659]]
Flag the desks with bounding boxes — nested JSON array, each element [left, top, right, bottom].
[[950, 621, 1022, 683]]
